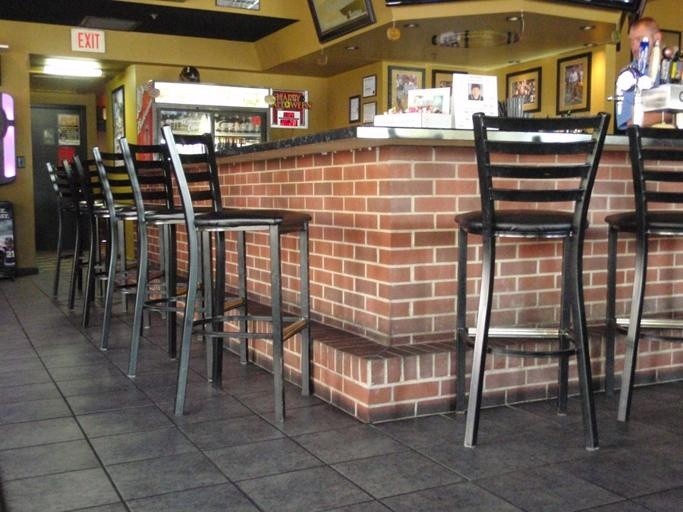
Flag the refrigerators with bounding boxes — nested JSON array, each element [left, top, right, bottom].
[[135, 80, 273, 161]]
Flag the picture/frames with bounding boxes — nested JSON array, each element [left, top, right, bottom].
[[430, 68, 468, 92], [306, 0, 376, 43], [110, 84, 125, 144], [385, 65, 425, 110], [348, 93, 361, 122], [505, 66, 541, 114], [361, 73, 376, 98], [555, 52, 592, 116], [362, 101, 377, 124]]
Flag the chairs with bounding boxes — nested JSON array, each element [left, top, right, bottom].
[[450, 109, 611, 452], [600, 123, 682, 421]]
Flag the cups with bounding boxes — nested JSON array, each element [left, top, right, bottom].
[[506, 97, 523, 118]]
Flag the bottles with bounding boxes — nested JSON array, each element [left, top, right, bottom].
[[213, 111, 259, 150], [159, 110, 210, 135]]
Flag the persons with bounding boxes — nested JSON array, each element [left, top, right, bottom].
[[615, 16, 672, 137], [397, 74, 417, 104], [566, 65, 583, 102], [513, 80, 536, 104], [468, 84, 483, 101]]
